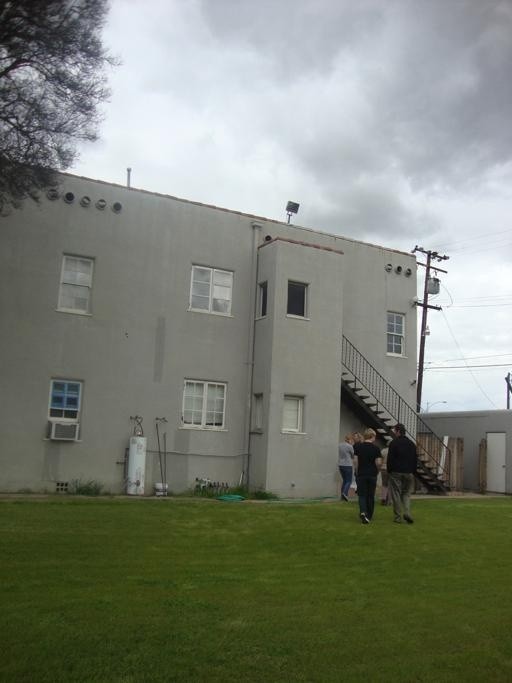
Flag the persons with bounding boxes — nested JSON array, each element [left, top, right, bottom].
[[337, 432, 355, 502], [387, 424, 417, 524], [352, 432, 365, 493], [379, 437, 395, 505], [354, 427, 383, 523]]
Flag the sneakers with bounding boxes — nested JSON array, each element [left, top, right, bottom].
[[360, 512, 369, 524], [402, 514, 414, 524], [341, 493, 349, 503]]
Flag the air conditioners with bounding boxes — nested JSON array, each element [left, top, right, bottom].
[[49, 420, 79, 442]]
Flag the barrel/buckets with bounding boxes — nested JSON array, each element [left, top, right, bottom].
[[155, 483, 168, 496]]
